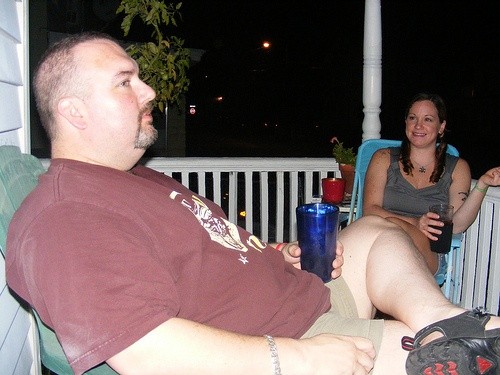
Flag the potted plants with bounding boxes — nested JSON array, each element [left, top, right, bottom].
[[331, 137, 355, 194]]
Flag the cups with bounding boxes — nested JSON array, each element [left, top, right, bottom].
[[322, 177, 346, 206], [295, 202, 340, 285], [428, 203, 454, 254]]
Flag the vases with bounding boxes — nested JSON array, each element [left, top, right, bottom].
[[322, 178, 344, 205]]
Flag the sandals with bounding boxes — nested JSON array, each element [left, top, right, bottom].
[[401, 306, 500, 375]]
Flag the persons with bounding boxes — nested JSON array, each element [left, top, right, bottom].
[[361, 94, 500, 284], [6, 30, 500, 375]]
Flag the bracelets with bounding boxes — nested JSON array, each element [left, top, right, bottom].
[[475, 184, 488, 192], [276, 242, 286, 251], [263, 334, 282, 375]]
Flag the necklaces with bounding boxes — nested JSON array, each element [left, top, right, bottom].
[[410, 156, 434, 172]]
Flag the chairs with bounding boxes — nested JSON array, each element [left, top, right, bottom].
[[342, 139, 463, 307], [0, 144, 118, 375]]
